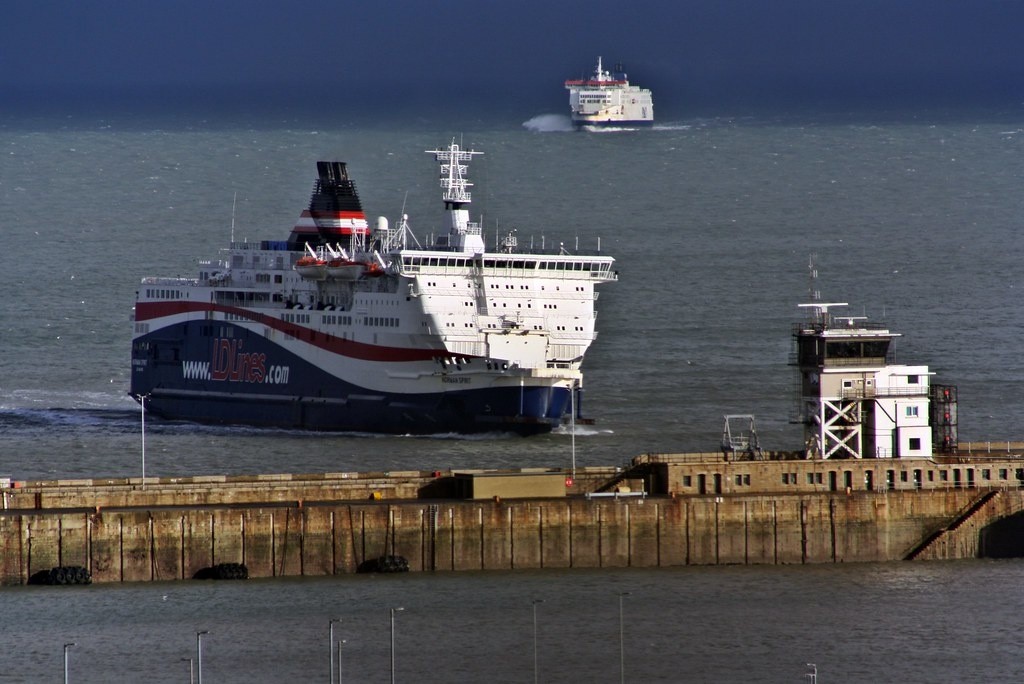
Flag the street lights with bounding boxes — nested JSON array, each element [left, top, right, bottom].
[[133, 392, 153, 492], [565, 382, 585, 479]]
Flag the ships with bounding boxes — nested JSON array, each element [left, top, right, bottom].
[[128, 133, 619, 436]]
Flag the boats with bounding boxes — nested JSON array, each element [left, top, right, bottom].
[[295, 256, 327, 279], [563, 55, 655, 130], [326, 259, 367, 280]]
